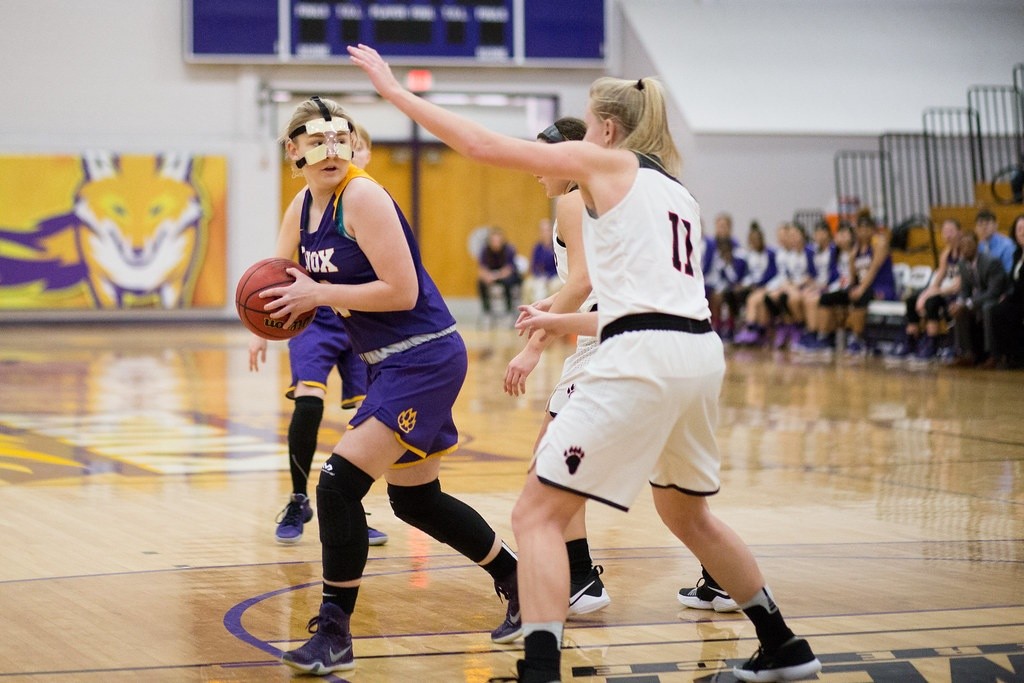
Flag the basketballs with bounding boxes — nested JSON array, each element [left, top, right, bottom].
[[234, 258, 319, 342]]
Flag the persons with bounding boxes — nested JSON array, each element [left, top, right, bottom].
[[476, 208, 1023, 369], [346, 43, 822, 683], [250, 126, 388, 546], [257, 96, 523, 674], [503, 116, 741, 616]]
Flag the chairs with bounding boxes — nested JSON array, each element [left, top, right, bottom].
[[490, 263, 937, 359]]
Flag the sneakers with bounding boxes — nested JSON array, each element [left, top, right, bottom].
[[724, 322, 961, 366], [733, 638, 821, 683], [491, 594, 520, 646], [368, 524, 383, 543], [280, 630, 355, 676], [678, 582, 741, 615], [567, 564, 611, 617], [276, 494, 314, 545]]
